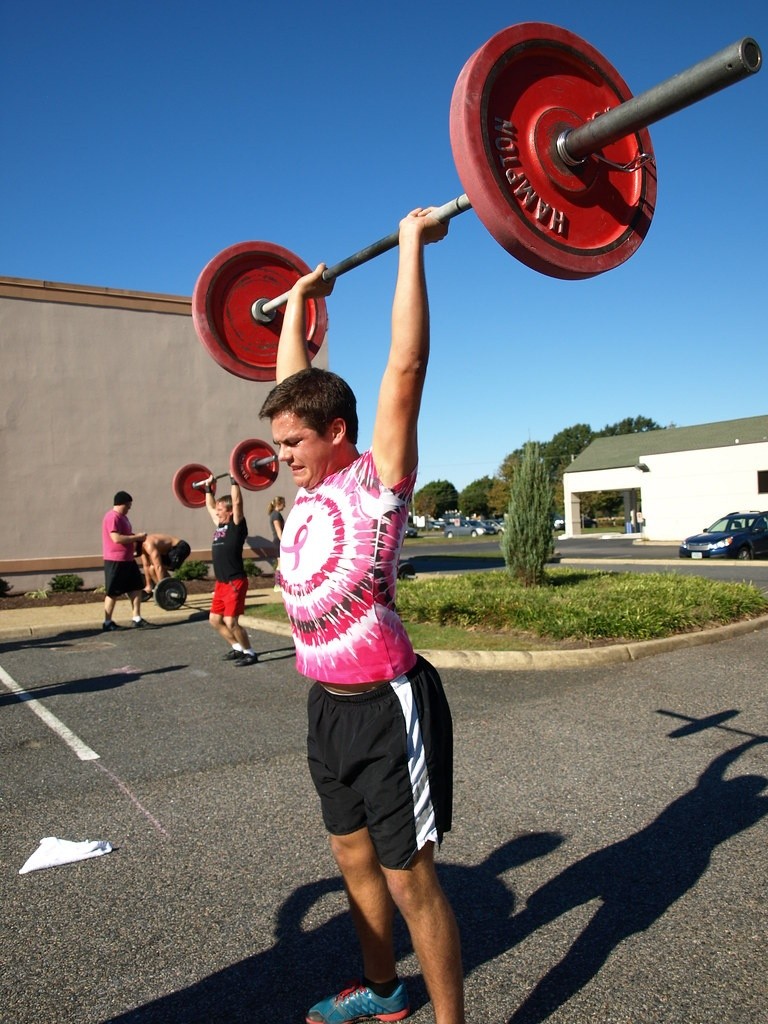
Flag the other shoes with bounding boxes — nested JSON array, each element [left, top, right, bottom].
[[131, 620, 161, 628], [103, 621, 127, 631]]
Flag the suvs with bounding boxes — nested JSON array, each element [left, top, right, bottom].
[[553, 515, 565, 531], [678, 507, 767, 561]]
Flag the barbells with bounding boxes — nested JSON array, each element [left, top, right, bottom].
[[171, 438, 280, 509], [189, 18, 764, 385], [125, 572, 188, 612]]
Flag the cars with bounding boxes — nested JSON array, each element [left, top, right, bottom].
[[444, 520, 495, 538], [483, 520, 507, 535], [428, 520, 447, 531], [404, 525, 418, 538]]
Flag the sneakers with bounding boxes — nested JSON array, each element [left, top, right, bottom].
[[223, 650, 243, 660], [307, 979, 409, 1024], [234, 654, 257, 666]]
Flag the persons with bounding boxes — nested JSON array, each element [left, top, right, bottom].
[[131, 533, 191, 593], [204, 470, 259, 667], [101, 491, 154, 631], [255, 206, 466, 1023], [266, 496, 286, 571]]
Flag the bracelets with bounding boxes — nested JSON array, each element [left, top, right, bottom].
[[230, 477, 239, 485], [205, 485, 211, 493]]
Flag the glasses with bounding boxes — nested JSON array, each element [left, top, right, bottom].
[[126, 503, 131, 506]]
